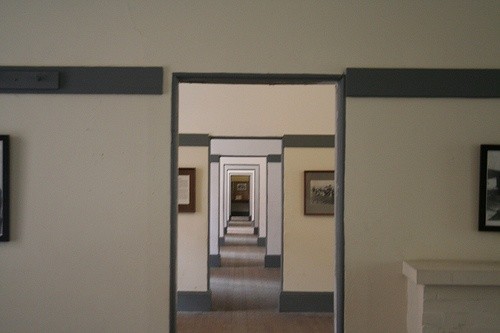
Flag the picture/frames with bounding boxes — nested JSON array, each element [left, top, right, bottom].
[[0, 134, 10, 242], [304, 170, 335, 215], [478, 144, 500, 232], [178, 167, 196, 212]]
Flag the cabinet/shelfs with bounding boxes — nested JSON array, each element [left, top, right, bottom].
[[401, 260, 500, 333]]
